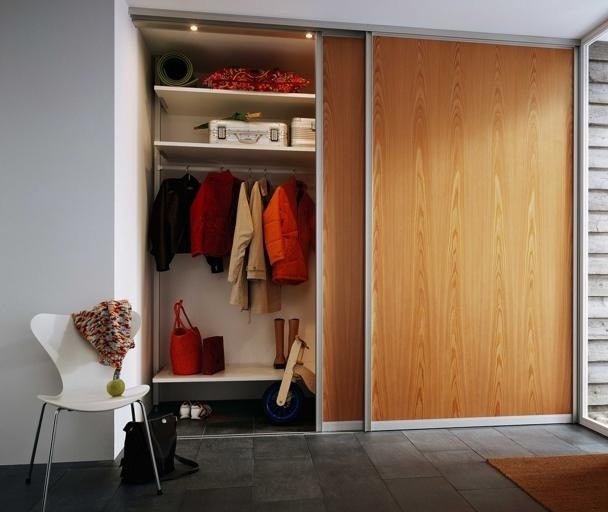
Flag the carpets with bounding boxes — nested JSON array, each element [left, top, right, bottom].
[[486, 453, 608, 512]]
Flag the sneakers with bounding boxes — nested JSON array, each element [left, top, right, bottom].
[[180, 401, 191, 421], [192, 401, 212, 420]]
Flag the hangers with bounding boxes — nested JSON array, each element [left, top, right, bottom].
[[183, 165, 302, 183]]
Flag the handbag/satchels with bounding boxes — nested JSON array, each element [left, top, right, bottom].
[[120, 413, 177, 485], [170, 301, 200, 375]]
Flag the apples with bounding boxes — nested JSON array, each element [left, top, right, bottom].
[[107, 379, 125, 397]]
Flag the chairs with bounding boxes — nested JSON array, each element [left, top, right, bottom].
[[26, 309, 164, 512]]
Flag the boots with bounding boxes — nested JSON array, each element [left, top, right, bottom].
[[287, 319, 299, 364], [274, 318, 285, 368]]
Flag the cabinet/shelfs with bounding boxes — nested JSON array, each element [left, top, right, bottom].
[[133, 18, 581, 438]]
